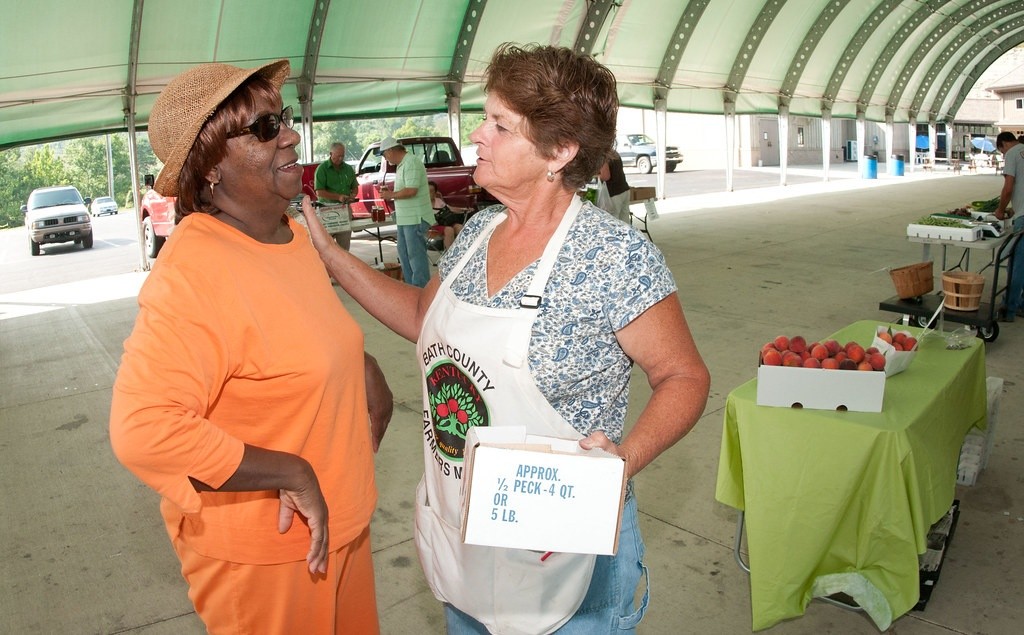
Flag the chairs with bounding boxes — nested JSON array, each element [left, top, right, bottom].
[[432, 150, 450, 163]]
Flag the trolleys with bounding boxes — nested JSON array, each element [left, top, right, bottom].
[[880, 229, 1023, 346]]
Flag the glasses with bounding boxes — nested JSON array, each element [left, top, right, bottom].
[[225, 106, 295, 142]]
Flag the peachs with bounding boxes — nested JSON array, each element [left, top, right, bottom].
[[877, 332, 918, 351], [761, 335, 886, 371]]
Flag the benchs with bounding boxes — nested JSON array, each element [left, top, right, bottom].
[[923, 166, 1002, 176]]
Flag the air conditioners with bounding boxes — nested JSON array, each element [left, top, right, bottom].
[[846, 140, 857, 160]]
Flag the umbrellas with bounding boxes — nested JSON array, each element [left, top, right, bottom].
[[971, 137, 996, 153], [916, 135, 929, 152]]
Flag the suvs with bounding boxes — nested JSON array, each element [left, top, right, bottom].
[[19, 185, 93, 256]]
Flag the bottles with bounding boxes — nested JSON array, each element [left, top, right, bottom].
[[371, 206, 385, 222]]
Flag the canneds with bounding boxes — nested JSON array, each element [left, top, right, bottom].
[[371, 206, 385, 223], [380, 185, 391, 201]]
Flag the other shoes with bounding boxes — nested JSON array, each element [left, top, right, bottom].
[[996, 307, 1024, 323]]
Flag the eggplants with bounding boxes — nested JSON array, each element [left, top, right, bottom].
[[946, 207, 972, 217]]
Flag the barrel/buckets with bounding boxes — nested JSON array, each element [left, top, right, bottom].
[[889, 261, 933, 299], [942, 271, 986, 311], [371, 262, 402, 282]]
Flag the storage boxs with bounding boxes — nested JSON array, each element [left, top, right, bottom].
[[634, 187, 657, 200], [459, 425, 629, 558], [906, 221, 983, 241], [952, 376, 1004, 487], [758, 351, 886, 413], [971, 210, 1012, 228]]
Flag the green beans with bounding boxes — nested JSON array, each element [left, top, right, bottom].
[[915, 217, 964, 227]]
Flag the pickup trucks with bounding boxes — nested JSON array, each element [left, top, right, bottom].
[[351, 136, 502, 219], [141, 163, 351, 258]]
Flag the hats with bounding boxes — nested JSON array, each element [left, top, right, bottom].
[[147, 58, 291, 197], [380, 137, 402, 151]]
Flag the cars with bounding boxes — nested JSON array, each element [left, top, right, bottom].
[[612, 134, 685, 174], [91, 197, 119, 217]]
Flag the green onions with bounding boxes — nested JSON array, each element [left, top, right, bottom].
[[966, 196, 1000, 212]]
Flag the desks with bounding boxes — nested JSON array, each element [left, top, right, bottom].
[[351, 216, 397, 264], [908, 224, 1016, 296], [628, 198, 659, 242], [729, 319, 989, 612], [969, 159, 1003, 175], [923, 158, 960, 175]]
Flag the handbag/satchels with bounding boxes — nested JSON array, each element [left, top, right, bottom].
[[596, 174, 615, 215], [434, 206, 456, 227]]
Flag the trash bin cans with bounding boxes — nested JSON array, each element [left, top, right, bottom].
[[864, 155, 877, 179], [891, 155, 904, 176]]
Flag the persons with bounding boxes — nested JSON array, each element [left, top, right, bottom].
[[600, 150, 630, 225], [996, 132, 1024, 322], [379, 136, 466, 288], [314, 143, 359, 284], [301, 42, 710, 635], [109, 59, 392, 635]]
